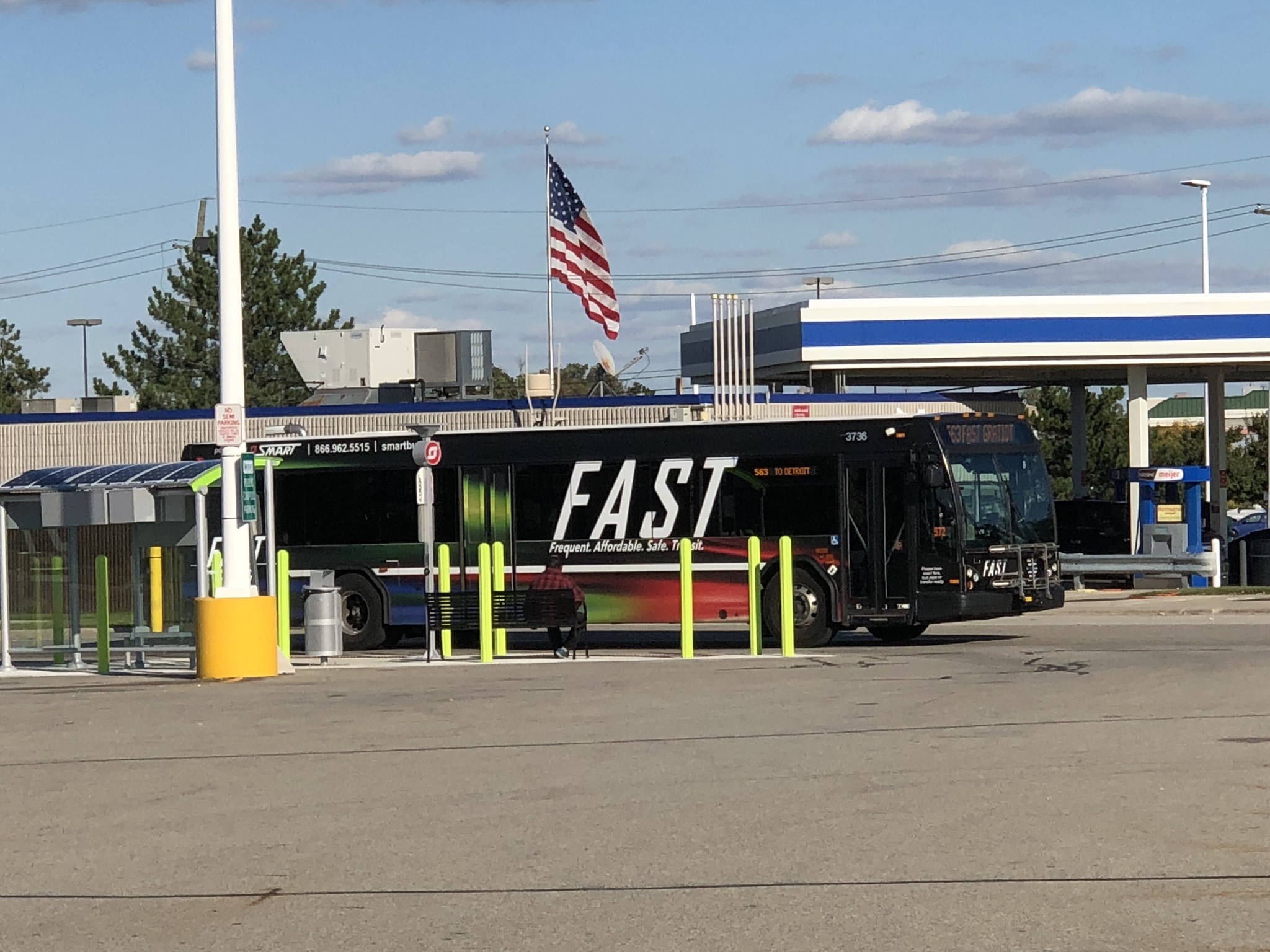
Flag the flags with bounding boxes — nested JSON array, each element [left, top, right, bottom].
[[547, 141, 620, 344]]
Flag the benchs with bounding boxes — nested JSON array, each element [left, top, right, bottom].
[[9, 631, 196, 673]]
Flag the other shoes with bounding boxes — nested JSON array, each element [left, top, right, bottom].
[[554, 649, 569, 658]]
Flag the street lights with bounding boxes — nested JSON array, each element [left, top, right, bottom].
[[66, 318, 103, 397], [1181, 178, 1223, 589]]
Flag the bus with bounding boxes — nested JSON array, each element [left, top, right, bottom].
[[179, 411, 1067, 651]]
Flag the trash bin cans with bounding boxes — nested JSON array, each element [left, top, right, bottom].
[[303, 570, 342, 666]]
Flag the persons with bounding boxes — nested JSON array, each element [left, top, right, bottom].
[[527, 553, 584, 659]]
